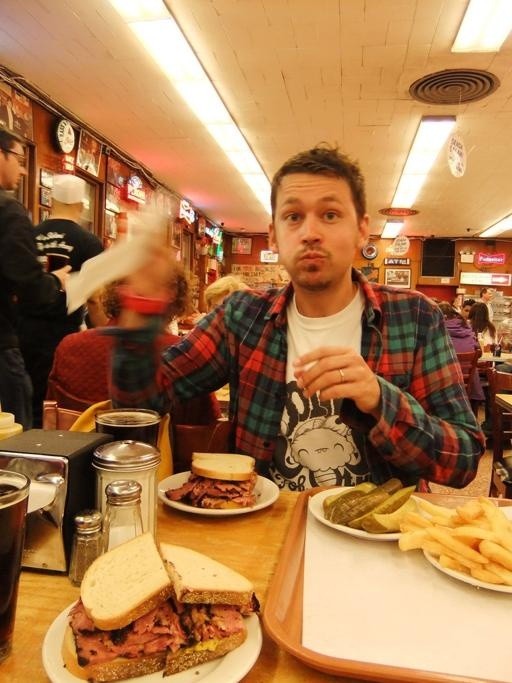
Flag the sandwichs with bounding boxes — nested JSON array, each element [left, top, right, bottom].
[[166, 443, 263, 511], [57, 530, 261, 681]]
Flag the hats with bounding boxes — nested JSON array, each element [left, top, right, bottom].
[[52, 174, 86, 204]]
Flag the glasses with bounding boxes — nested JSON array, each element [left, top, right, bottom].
[[2, 147, 24, 162]]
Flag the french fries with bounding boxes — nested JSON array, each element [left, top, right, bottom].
[[396, 494, 512, 585]]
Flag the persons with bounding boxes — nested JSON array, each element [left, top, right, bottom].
[[464, 301, 498, 359], [109, 139, 483, 507], [432, 300, 475, 356], [39, 238, 226, 426], [478, 287, 494, 324], [28, 172, 101, 342], [459, 299, 476, 321], [201, 276, 261, 404], [1, 125, 76, 430]]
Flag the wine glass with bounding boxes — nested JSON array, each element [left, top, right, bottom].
[[477, 333, 484, 358]]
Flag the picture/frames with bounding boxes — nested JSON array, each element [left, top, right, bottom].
[[108, 156, 130, 188], [168, 221, 182, 249], [259, 249, 278, 263], [384, 267, 411, 288], [231, 237, 253, 255], [39, 167, 55, 226], [77, 127, 103, 178], [106, 185, 121, 212], [103, 212, 120, 240]]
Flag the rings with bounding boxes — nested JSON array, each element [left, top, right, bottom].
[[337, 367, 346, 383]]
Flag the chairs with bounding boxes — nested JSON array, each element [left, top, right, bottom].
[[454, 348, 512, 501], [39, 398, 232, 472]]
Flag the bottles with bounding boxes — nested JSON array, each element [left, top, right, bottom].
[[91, 440, 161, 543], [102, 479, 144, 552], [68, 510, 104, 586]]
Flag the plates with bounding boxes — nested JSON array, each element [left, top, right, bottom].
[[423, 507, 512, 593], [309, 486, 432, 542], [41, 600, 264, 682], [158, 471, 281, 517]]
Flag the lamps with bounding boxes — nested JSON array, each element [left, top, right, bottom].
[[449, 0, 511, 54], [387, 115, 457, 211], [380, 218, 404, 239], [109, 0, 273, 214], [477, 211, 512, 239]]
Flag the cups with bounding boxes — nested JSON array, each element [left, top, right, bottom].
[[95, 408, 160, 448], [0, 468, 31, 661], [494, 343, 502, 357]]
